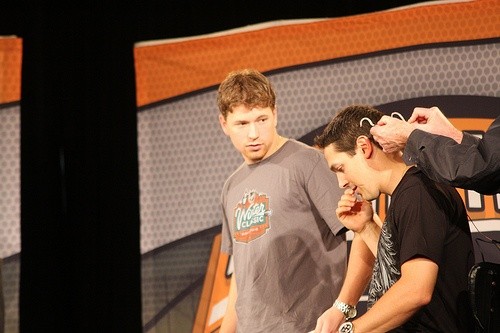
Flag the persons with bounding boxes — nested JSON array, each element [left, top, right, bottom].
[[369, 106, 500, 196], [215, 67, 383, 333], [314, 104, 476, 333]]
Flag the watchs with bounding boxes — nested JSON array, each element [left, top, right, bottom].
[[332, 298, 357, 319], [337, 322, 355, 333]]
